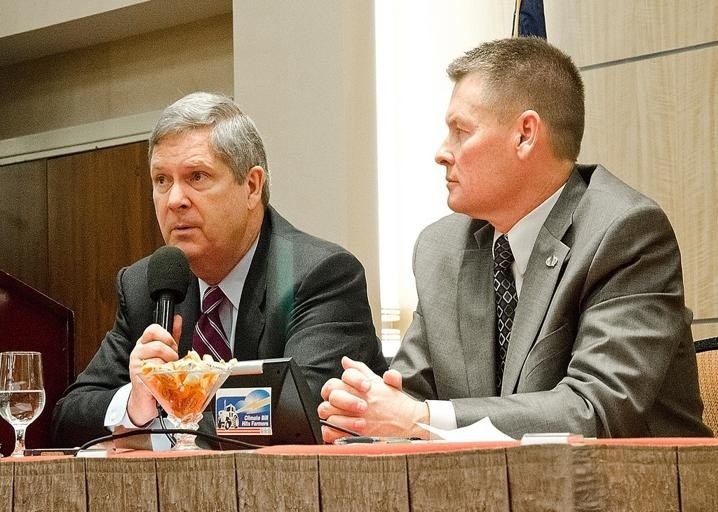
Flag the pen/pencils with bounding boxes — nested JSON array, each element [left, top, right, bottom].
[[335, 436, 425, 444]]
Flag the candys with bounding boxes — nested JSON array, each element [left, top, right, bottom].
[[141, 349, 236, 420]]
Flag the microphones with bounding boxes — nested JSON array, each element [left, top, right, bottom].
[[148, 245, 190, 419]]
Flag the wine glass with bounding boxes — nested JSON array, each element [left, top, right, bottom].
[[1, 351, 46, 459], [139, 366, 226, 452]]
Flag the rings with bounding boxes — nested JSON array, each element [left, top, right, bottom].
[[140, 359, 146, 368]]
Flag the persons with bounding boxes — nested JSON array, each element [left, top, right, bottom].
[[48, 90, 390, 448], [317, 36, 713, 443]]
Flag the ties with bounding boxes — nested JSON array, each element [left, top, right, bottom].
[[491, 235, 518, 396], [191, 283, 231, 363]]
